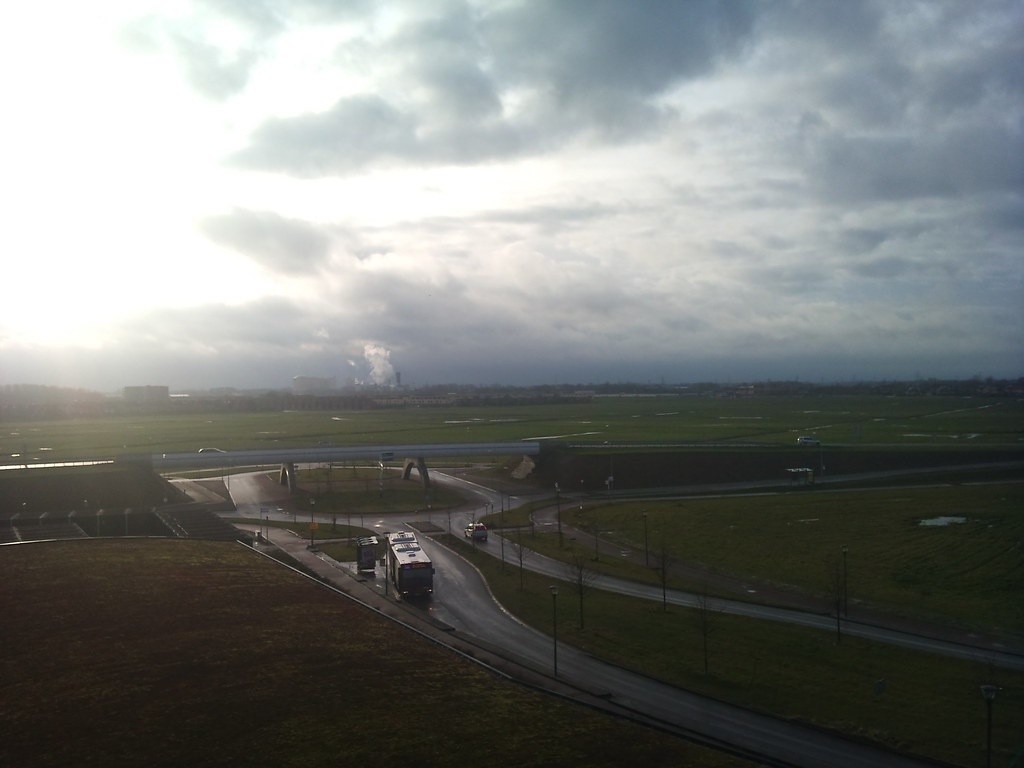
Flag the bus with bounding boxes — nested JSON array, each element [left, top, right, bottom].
[[386, 531, 436, 602]]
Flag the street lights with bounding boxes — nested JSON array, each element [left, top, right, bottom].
[[841, 543, 850, 630], [642, 508, 648, 567], [555, 480, 564, 547], [981, 684, 997, 767], [547, 584, 559, 681], [310, 499, 316, 550]]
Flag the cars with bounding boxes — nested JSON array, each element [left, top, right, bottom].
[[795, 437, 822, 446], [463, 520, 488, 541], [197, 446, 229, 453]]
